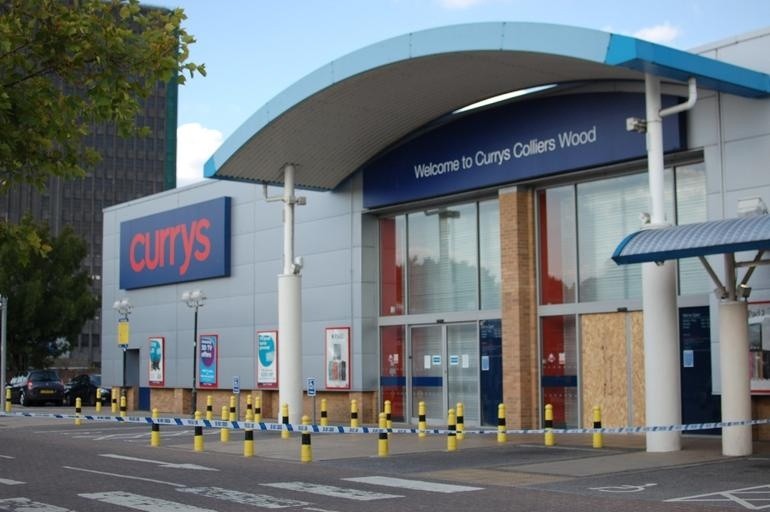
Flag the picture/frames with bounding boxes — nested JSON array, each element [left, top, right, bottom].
[[255, 329, 279, 388], [148, 337, 164, 386], [324, 326, 354, 389], [197, 334, 219, 387]]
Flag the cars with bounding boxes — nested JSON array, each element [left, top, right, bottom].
[[6, 371, 109, 407]]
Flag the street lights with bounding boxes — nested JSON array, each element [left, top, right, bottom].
[[183, 288, 208, 413], [113, 300, 130, 413]]
